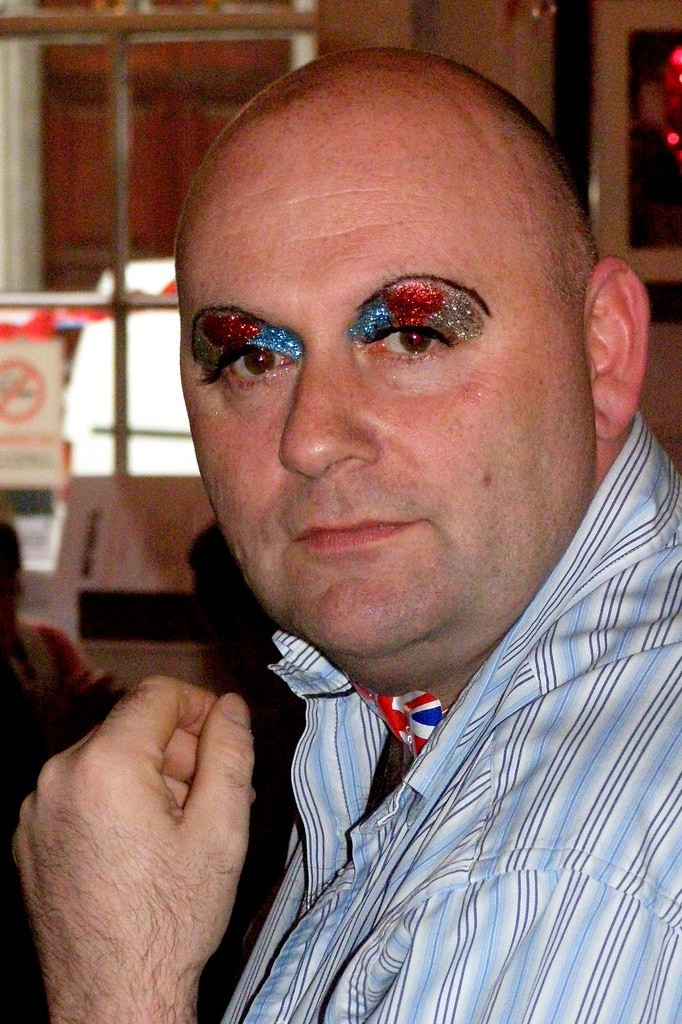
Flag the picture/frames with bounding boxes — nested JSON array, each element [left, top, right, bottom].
[[551, 0, 682, 325]]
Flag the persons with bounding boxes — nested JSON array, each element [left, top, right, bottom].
[[0, 523, 303, 758], [13, 47, 682, 1024]]
